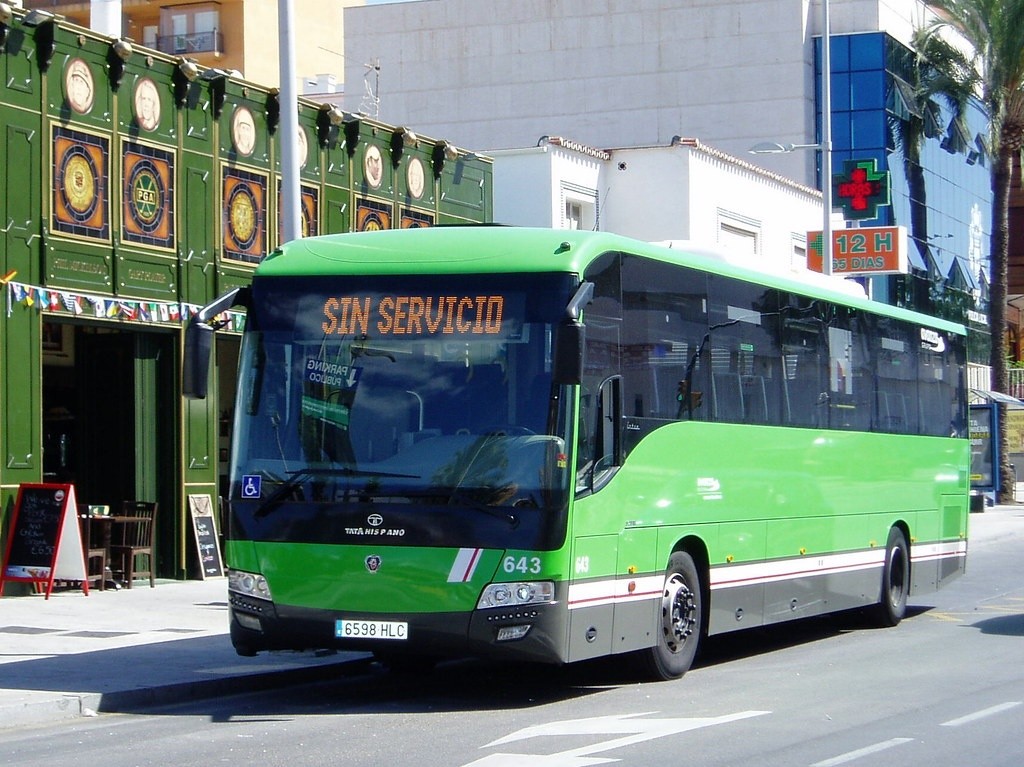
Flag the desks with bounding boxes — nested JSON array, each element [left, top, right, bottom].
[[82, 514, 152, 590]]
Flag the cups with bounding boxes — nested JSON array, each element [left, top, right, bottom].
[[89, 505, 98, 515], [98, 505, 109, 516]]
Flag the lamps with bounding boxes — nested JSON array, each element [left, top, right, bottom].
[[321, 103, 343, 125], [21, 10, 54, 28], [0, 3, 13, 27], [395, 126, 416, 148], [178, 56, 198, 82], [113, 38, 133, 60], [436, 140, 458, 161], [462, 151, 484, 161], [198, 68, 230, 80], [342, 111, 364, 124]]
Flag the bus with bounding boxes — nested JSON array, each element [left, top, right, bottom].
[[178, 224, 972, 683]]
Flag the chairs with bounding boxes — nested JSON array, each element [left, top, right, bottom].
[[905, 395, 926, 432], [871, 391, 891, 430], [58, 504, 106, 592], [887, 392, 909, 432], [713, 371, 745, 420], [93, 501, 158, 588], [426, 361, 471, 434], [653, 366, 718, 420], [739, 375, 769, 421], [764, 378, 791, 423], [467, 363, 508, 428]]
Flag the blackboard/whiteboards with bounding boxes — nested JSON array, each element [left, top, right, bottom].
[[194, 515, 224, 579], [5, 485, 68, 582]]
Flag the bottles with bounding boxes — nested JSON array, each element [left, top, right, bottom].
[[223, 408, 228, 420]]
